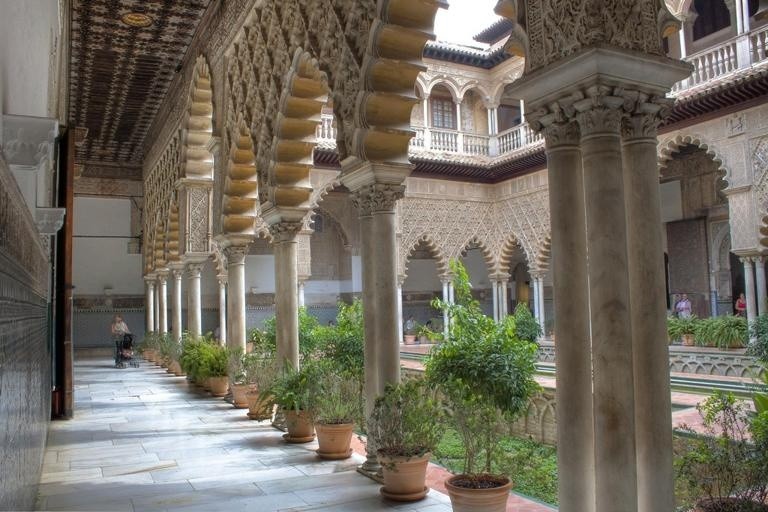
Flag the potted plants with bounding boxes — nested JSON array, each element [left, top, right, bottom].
[[663, 309, 749, 352], [367, 373, 447, 496], [307, 366, 362, 454], [674, 384, 768, 512], [138, 327, 279, 419], [418, 254, 542, 512], [246, 360, 337, 436], [403, 325, 445, 346]]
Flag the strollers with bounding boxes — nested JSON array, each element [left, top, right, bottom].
[[114, 333, 140, 370]]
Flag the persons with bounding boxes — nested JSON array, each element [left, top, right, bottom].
[[404, 314, 415, 332], [733, 291, 748, 315], [676, 292, 692, 320], [212, 324, 221, 340], [110, 313, 132, 369]]
